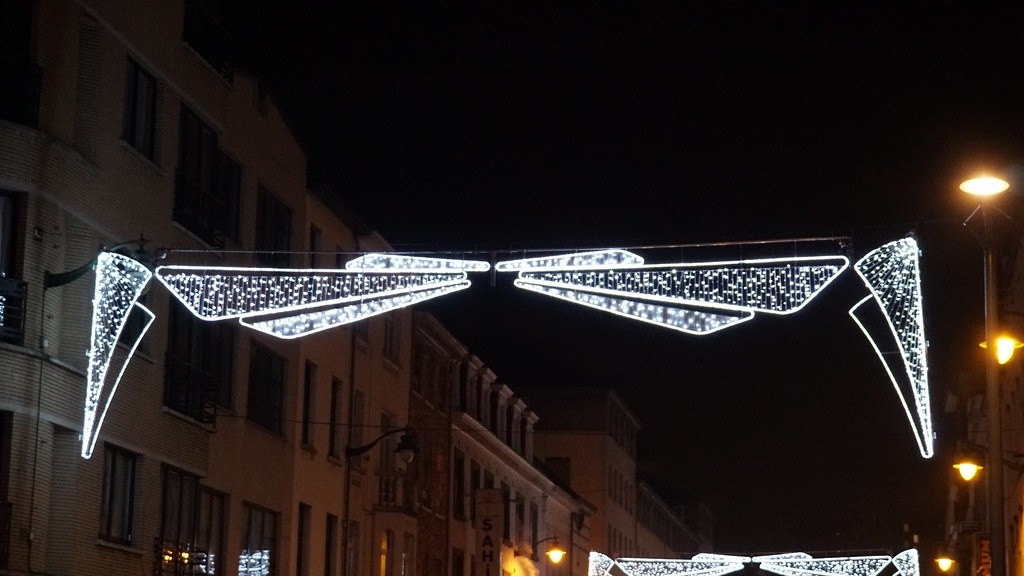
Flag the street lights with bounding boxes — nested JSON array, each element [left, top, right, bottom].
[[960, 159, 1009, 576]]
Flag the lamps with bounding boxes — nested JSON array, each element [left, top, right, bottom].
[[46, 233, 154, 302], [527, 536, 568, 563], [346, 426, 422, 464], [953, 442, 1024, 482]]
